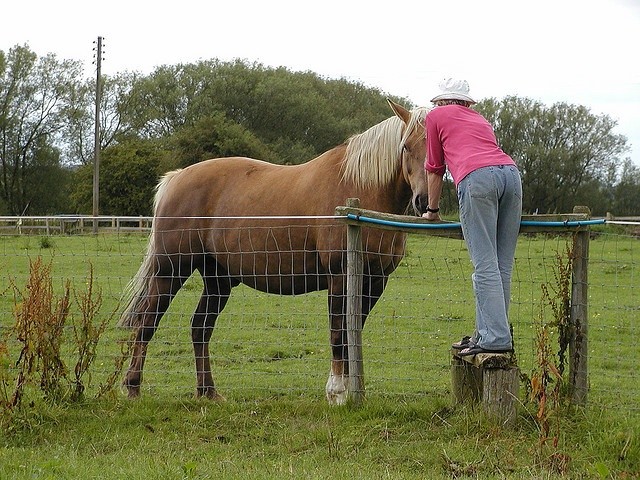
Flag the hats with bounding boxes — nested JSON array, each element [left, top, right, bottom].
[[431, 76, 477, 106]]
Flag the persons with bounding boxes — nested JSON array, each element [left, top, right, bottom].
[[423, 74, 526, 357]]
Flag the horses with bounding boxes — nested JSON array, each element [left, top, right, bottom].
[[117, 97, 432, 407]]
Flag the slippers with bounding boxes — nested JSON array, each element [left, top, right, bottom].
[[451, 336, 477, 349], [457, 343, 505, 356]]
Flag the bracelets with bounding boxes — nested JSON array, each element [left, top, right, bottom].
[[425, 205, 441, 215]]
